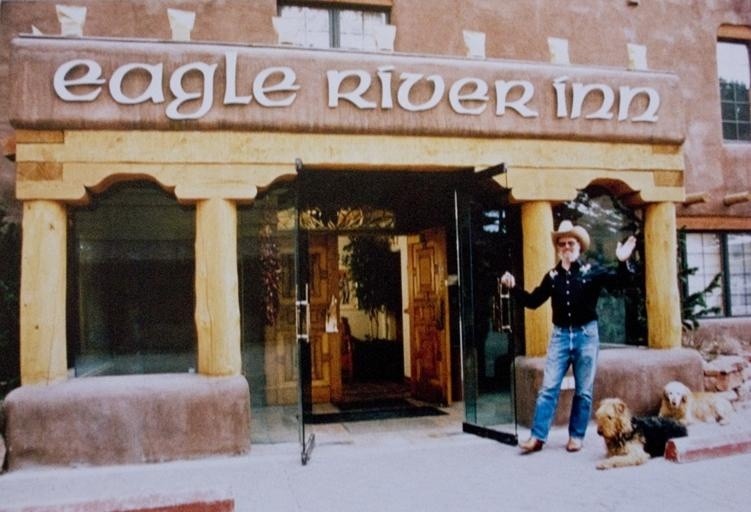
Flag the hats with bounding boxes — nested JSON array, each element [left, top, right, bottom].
[[550, 219, 590, 252]]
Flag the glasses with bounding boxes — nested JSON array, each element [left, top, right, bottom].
[[557, 240, 580, 246]]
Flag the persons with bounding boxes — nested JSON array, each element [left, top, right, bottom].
[[500, 219, 640, 451]]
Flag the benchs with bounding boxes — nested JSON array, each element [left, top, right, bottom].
[[511, 347, 702, 438], [703, 355, 751, 402], [0, 374, 249, 470]]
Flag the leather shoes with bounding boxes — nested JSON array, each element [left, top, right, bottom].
[[567, 437, 583, 451], [520, 437, 543, 451]]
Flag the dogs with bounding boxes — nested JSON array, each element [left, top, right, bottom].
[[594, 395, 689, 471], [658, 380, 735, 427]]
